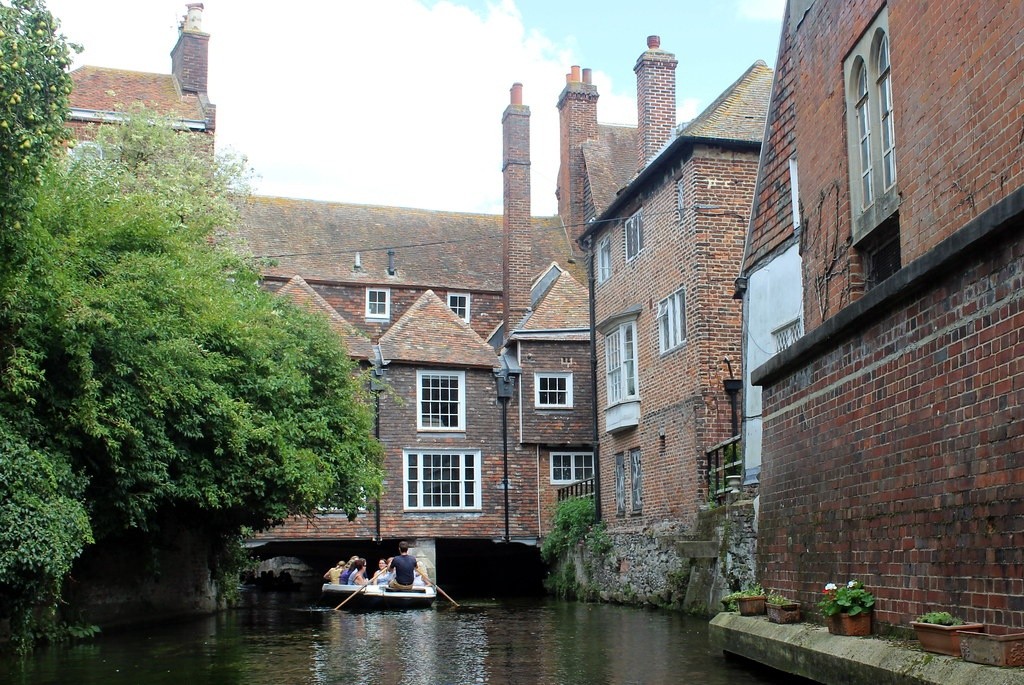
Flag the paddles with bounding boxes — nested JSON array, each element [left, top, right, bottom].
[[334, 568, 388, 611], [413, 568, 461, 608]]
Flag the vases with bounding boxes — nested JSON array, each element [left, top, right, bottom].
[[827, 613, 871, 635], [956, 629, 1024, 667]]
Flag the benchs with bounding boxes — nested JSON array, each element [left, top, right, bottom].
[[386, 588, 426, 592]]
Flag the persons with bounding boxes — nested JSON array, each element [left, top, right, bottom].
[[324, 561, 345, 584], [373, 557, 432, 587], [339, 556, 371, 587], [388, 542, 418, 590]]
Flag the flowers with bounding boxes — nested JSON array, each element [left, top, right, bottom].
[[817, 578, 874, 616]]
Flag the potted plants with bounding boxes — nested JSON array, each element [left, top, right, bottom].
[[909, 611, 984, 656], [765, 590, 801, 623], [739, 582, 766, 616], [724, 442, 742, 494]]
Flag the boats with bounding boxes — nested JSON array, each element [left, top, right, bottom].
[[318, 583, 438, 613]]
